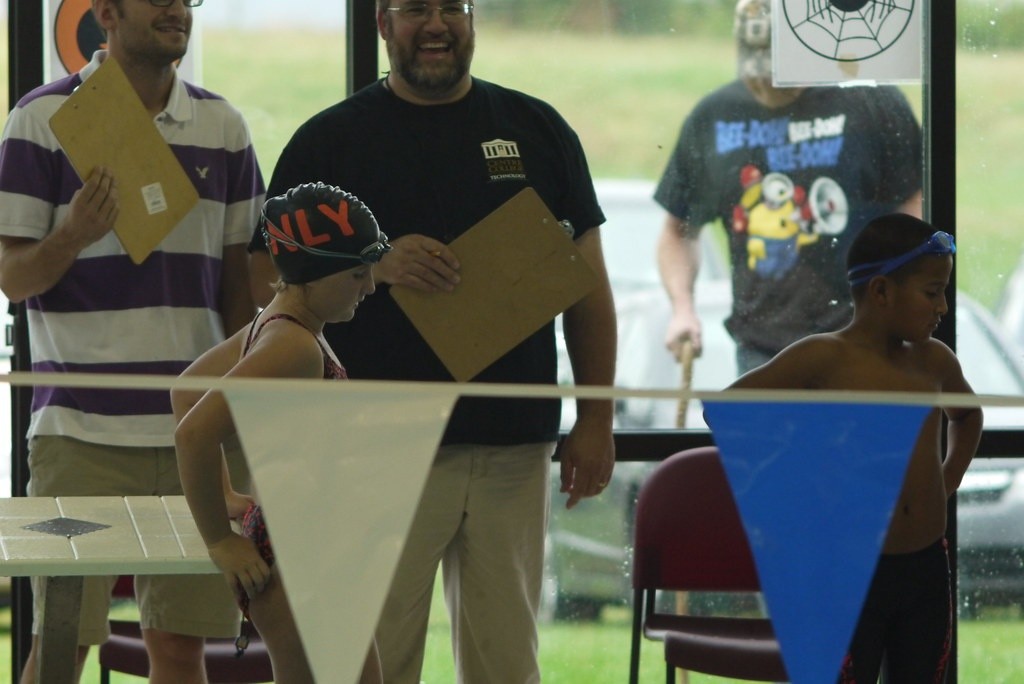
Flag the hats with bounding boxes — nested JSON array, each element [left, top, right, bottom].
[[262, 181, 382, 284], [734, 0, 773, 77]]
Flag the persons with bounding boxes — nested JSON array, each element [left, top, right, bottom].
[[0, 0, 266, 683], [651, 0, 923, 378], [707, 212, 984, 684], [263, 0, 617, 684], [168, 183, 391, 684]]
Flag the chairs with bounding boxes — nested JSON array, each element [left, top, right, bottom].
[[629, 443, 792, 684], [99, 569, 273, 684]]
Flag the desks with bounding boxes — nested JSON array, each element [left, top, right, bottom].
[[0, 496, 266, 684]]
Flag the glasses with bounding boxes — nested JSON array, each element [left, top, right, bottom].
[[388, 2, 476, 19], [148, 0, 203, 8], [357, 231, 392, 266], [877, 230, 958, 275]]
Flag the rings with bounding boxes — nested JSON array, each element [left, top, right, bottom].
[[597, 482, 608, 488]]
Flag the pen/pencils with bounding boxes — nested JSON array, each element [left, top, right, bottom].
[[431, 251, 440, 257]]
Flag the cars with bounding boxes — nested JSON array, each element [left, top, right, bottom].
[[538, 177, 1024, 626]]
